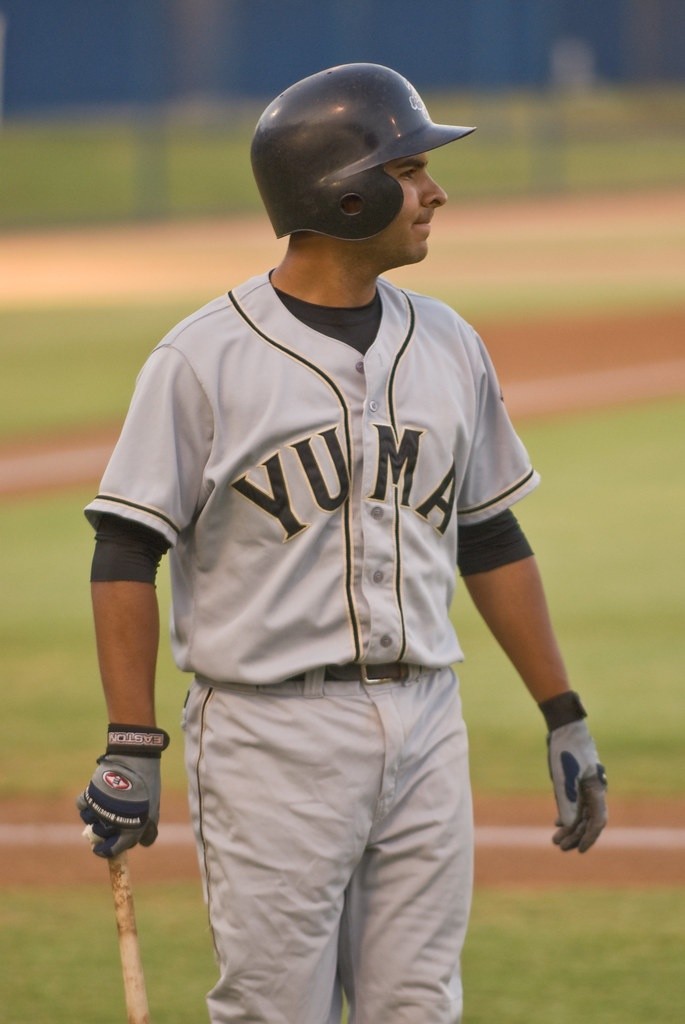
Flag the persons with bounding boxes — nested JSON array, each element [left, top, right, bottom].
[[77, 63, 607, 1024]]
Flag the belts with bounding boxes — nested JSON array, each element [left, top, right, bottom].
[[287, 663, 401, 684]]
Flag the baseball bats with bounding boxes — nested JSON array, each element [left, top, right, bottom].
[[107, 851, 152, 1024]]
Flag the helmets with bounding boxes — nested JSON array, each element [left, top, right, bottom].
[[250, 62, 478, 241]]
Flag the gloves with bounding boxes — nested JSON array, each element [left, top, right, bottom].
[[539, 691, 608, 854], [76, 724, 171, 858]]
